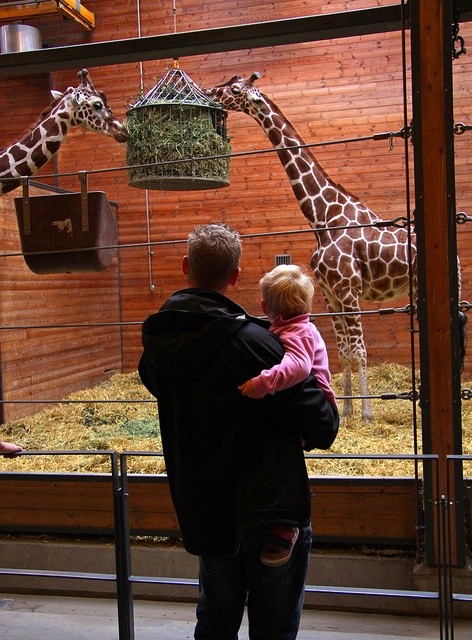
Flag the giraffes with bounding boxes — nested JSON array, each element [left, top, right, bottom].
[[202, 71, 462, 425], [0, 68, 129, 197]]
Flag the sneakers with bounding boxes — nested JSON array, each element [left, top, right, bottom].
[[261, 526, 299, 567]]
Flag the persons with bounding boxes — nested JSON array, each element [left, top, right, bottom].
[[237, 262, 341, 568], [136, 221, 340, 640]]
